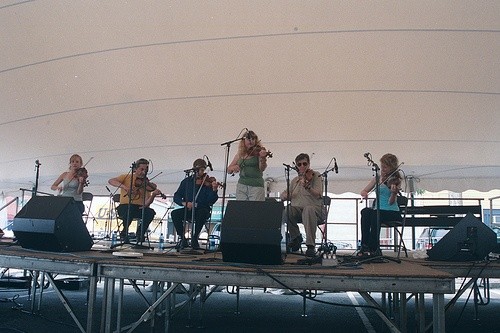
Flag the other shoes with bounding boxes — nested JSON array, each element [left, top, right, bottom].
[[357, 246, 382, 256], [135, 230, 145, 241], [122, 229, 128, 237], [175, 238, 199, 250]]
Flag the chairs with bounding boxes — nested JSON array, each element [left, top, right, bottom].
[[295, 195, 332, 255], [185, 205, 214, 251], [380, 195, 409, 260], [112, 194, 151, 247], [78, 192, 94, 225]]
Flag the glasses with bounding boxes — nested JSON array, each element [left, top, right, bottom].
[[296, 162, 309, 166]]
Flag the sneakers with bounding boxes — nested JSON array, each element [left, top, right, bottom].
[[292, 236, 303, 251], [305, 245, 316, 255]]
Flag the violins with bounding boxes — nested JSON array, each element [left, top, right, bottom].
[[248, 145, 273, 158], [135, 178, 166, 199], [196, 173, 225, 189], [382, 171, 401, 192], [303, 169, 314, 190], [75, 167, 90, 187]]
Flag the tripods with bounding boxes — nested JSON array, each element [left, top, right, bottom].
[[274, 164, 343, 266], [351, 152, 402, 268], [159, 164, 207, 253], [110, 166, 154, 251]]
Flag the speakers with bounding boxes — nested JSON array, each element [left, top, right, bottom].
[[12, 195, 94, 252], [425, 211, 497, 262], [220, 200, 286, 265]]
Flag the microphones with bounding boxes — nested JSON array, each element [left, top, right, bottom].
[[34, 160, 39, 171], [143, 162, 149, 175], [293, 162, 301, 173], [133, 162, 136, 173], [371, 161, 381, 171], [208, 160, 213, 171], [334, 161, 338, 174], [246, 130, 253, 145]]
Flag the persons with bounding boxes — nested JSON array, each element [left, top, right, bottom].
[[50, 154, 86, 217], [281, 152, 327, 257], [227, 131, 268, 202], [171, 158, 220, 249], [108, 159, 161, 242], [356, 153, 402, 258]]
